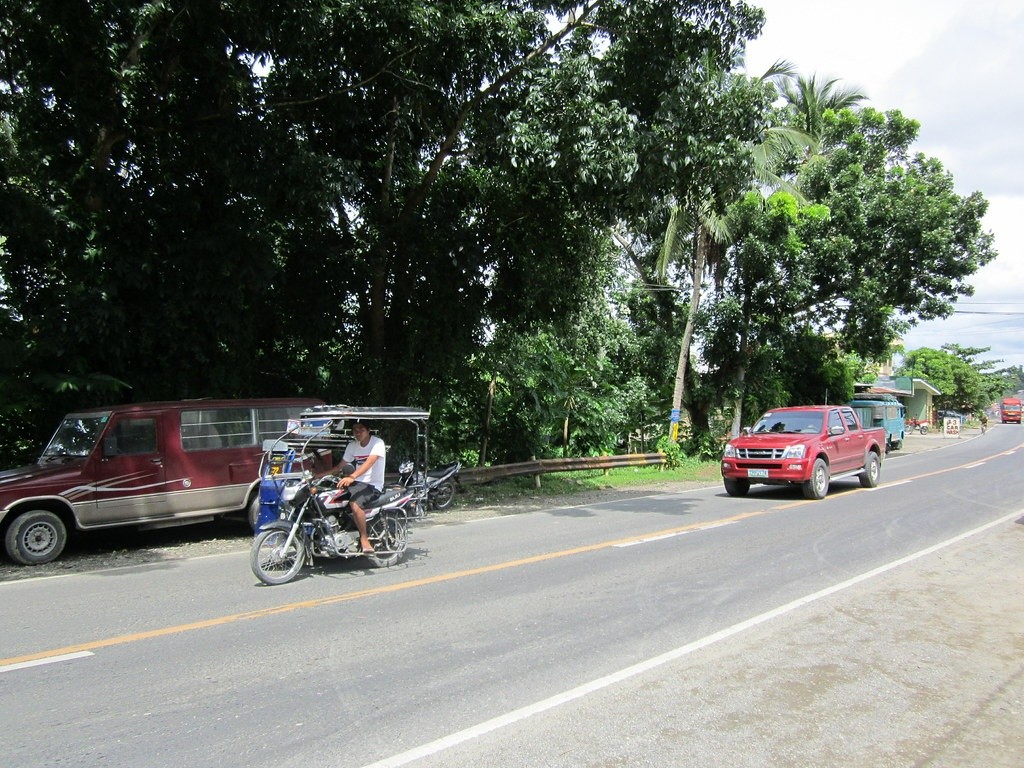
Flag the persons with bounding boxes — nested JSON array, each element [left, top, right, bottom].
[[981, 415, 988, 434], [312, 420, 386, 553]]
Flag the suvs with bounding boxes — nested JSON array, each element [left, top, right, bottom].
[[1, 393, 334, 567]]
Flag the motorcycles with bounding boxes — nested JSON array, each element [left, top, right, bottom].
[[903, 415, 930, 435], [398, 457, 463, 511], [248, 404, 432, 586]]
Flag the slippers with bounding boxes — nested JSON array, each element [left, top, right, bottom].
[[362, 547, 375, 552]]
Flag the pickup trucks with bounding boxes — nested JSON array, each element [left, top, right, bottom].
[[719, 404, 887, 499]]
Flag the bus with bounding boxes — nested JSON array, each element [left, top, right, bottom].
[[1001, 398, 1023, 424]]
